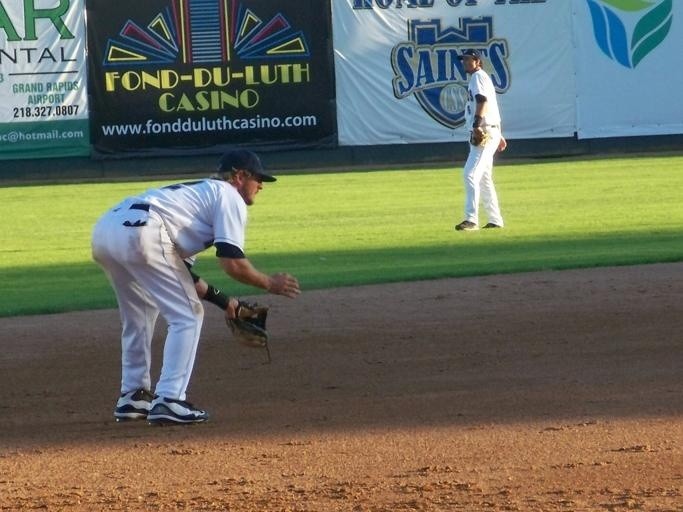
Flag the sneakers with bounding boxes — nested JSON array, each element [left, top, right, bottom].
[[146, 393, 209, 423], [113, 387, 155, 420], [455, 221, 479, 230], [482, 223, 501, 228]]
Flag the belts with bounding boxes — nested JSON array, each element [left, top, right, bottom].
[[130, 203, 150, 212]]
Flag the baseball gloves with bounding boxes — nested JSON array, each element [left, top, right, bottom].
[[470, 128, 486, 145], [225, 301, 268, 348]]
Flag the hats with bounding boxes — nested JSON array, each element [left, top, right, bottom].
[[456, 48, 483, 60], [216, 148, 277, 182]]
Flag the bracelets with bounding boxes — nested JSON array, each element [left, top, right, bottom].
[[203, 284, 230, 310], [472, 114, 482, 128]]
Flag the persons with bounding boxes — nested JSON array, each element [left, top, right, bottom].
[[455, 49, 507, 231], [91, 148, 302, 426]]
